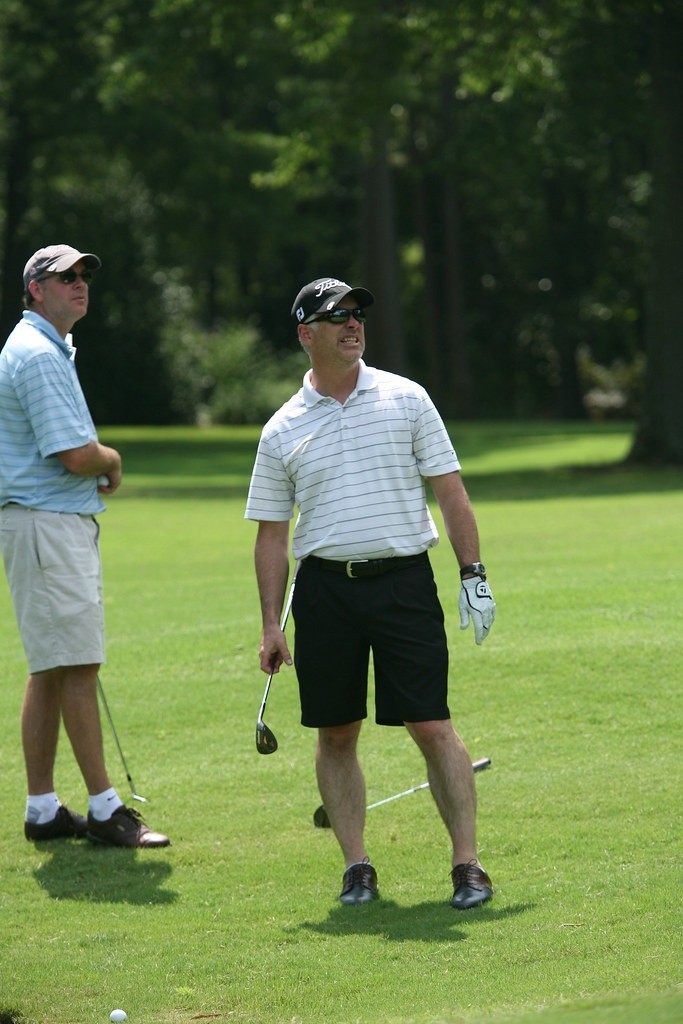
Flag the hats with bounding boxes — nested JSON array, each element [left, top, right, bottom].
[[291, 278, 375, 326], [22, 243, 101, 289]]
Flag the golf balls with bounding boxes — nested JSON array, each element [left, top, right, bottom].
[[109, 1009, 127, 1023]]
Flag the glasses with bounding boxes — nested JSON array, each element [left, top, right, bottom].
[[303, 307, 366, 324], [37, 268, 93, 285]]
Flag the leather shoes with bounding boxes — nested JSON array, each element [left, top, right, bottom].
[[340, 856, 377, 905], [449, 859, 493, 909]]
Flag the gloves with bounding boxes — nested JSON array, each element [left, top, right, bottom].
[[458, 575, 496, 646]]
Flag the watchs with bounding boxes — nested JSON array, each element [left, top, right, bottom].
[[460, 562, 488, 580]]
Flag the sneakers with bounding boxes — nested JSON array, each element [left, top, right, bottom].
[[25, 804, 87, 842], [85, 805, 172, 849]]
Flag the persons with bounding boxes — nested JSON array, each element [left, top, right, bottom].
[[0, 244, 171, 848], [244, 278, 498, 909]]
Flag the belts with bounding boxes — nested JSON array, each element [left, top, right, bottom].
[[301, 550, 429, 579]]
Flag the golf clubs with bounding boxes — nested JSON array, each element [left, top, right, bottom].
[[96, 675, 146, 802], [255, 560, 304, 755], [313, 757, 494, 829]]
